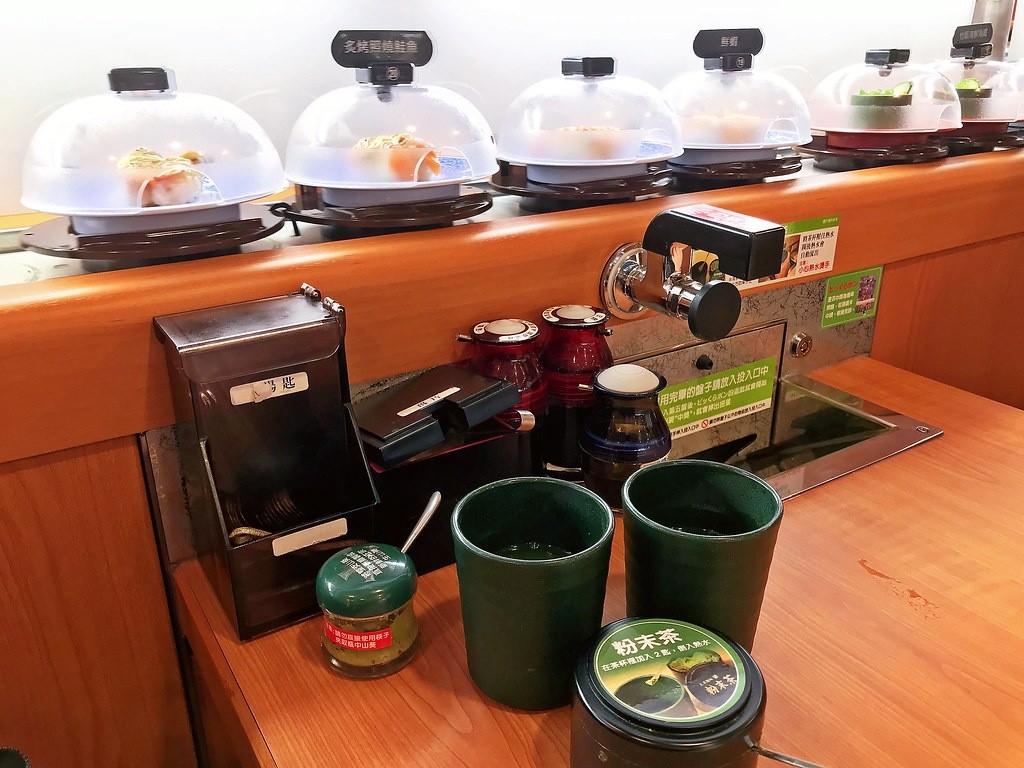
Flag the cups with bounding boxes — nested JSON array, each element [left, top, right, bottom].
[[666, 650, 723, 685], [450, 477, 615, 711], [570, 613, 767, 768], [614, 675, 697, 719], [622, 459, 784, 654]]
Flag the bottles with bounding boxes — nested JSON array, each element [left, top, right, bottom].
[[315, 542, 422, 681]]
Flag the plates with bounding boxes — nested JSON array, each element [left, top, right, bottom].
[[285, 157, 502, 208], [17, 157, 289, 234], [943, 118, 1024, 137], [810, 119, 963, 148], [667, 131, 812, 166], [499, 138, 685, 184]]
